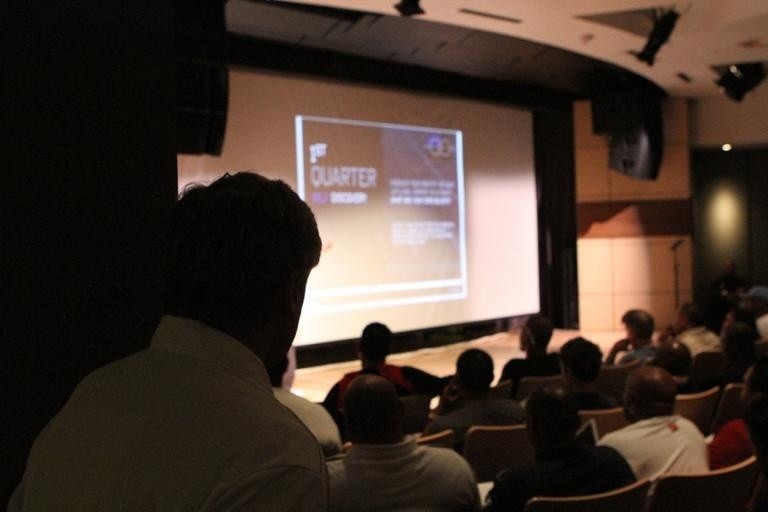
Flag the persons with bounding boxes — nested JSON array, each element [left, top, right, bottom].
[[747, 287, 768, 339], [739, 366, 767, 403], [656, 341, 692, 383], [328, 374, 482, 511], [420, 348, 527, 451], [28, 171, 331, 510], [601, 366, 711, 478], [710, 397, 766, 470], [485, 385, 636, 512], [607, 310, 674, 368], [659, 302, 719, 352], [721, 308, 758, 332], [712, 257, 743, 310], [499, 313, 559, 383], [692, 322, 759, 390], [322, 324, 451, 433], [555, 339, 618, 406]]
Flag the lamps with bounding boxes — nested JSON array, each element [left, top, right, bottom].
[[626, 8, 681, 65], [715, 62, 768, 102]]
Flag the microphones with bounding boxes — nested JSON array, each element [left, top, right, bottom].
[[668, 237, 684, 251]]
